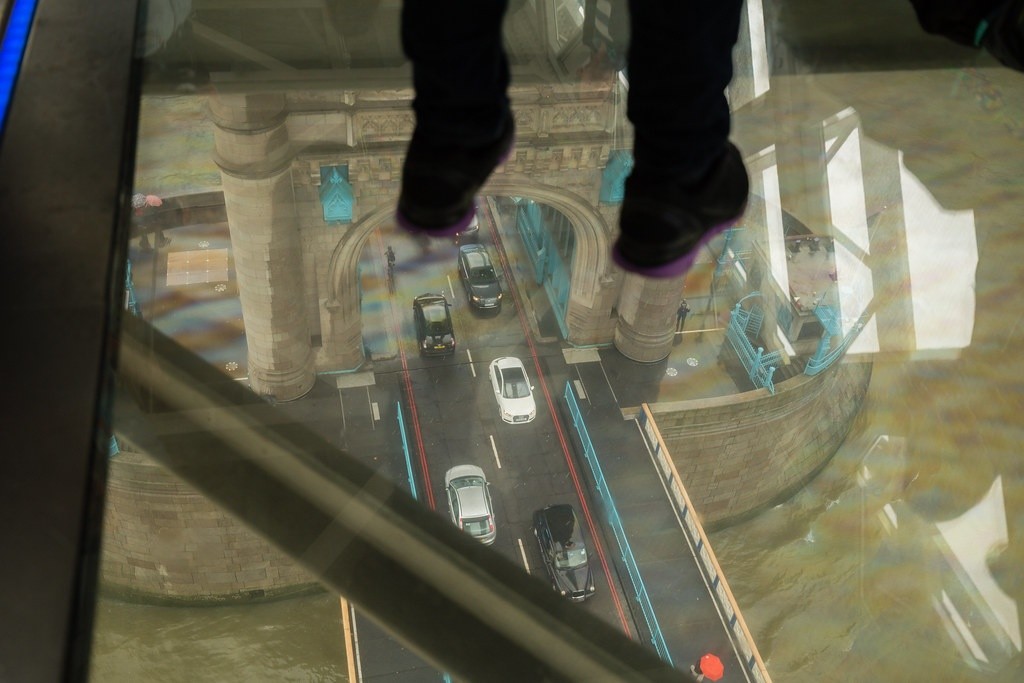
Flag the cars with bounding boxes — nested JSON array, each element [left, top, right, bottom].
[[531, 503, 596, 604], [488, 356, 537, 425], [457, 244, 503, 313], [445, 464, 497, 546], [413, 292, 457, 356], [453, 204, 481, 239]]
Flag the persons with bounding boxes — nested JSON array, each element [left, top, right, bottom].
[[793, 296, 804, 311], [395, 1, 747, 278], [383, 245, 396, 295], [676, 298, 691, 332]]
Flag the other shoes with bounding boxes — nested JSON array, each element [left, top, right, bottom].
[[396, 105, 515, 236], [612, 141, 750, 278]]
